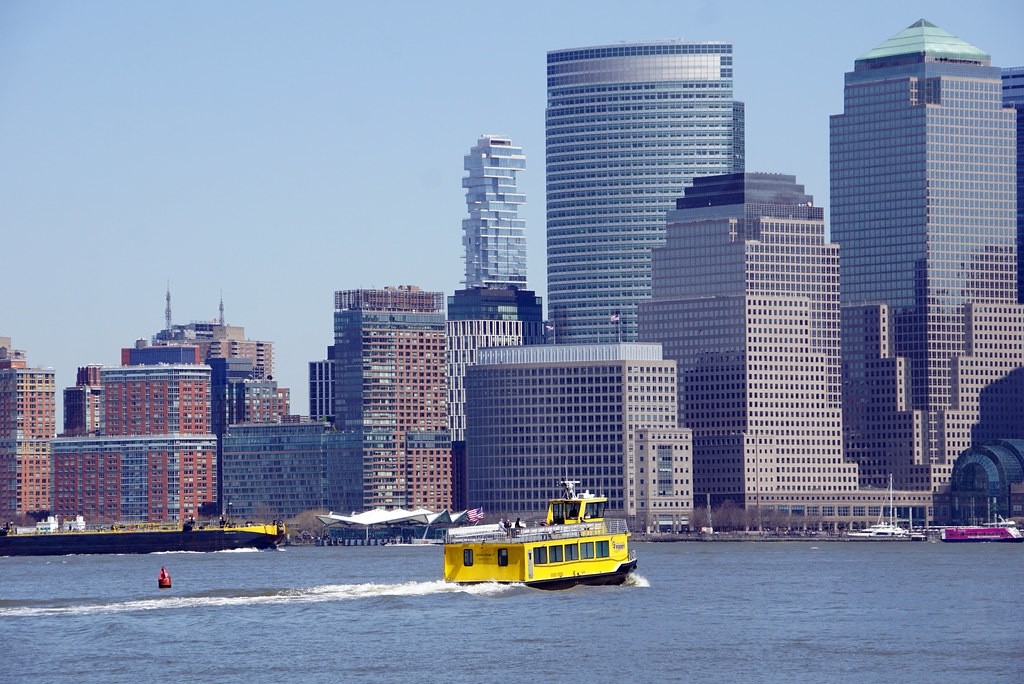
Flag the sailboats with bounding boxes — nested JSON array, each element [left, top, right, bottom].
[[841, 474, 928, 542]]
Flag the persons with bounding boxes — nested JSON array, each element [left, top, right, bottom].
[[504, 517, 586, 528]]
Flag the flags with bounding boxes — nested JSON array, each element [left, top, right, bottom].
[[611, 315, 619, 321], [467, 507, 484, 523], [546, 326, 553, 331]]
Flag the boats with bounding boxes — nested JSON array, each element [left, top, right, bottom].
[[938, 526, 1024, 543], [0, 517, 288, 557], [442, 477, 639, 590]]
